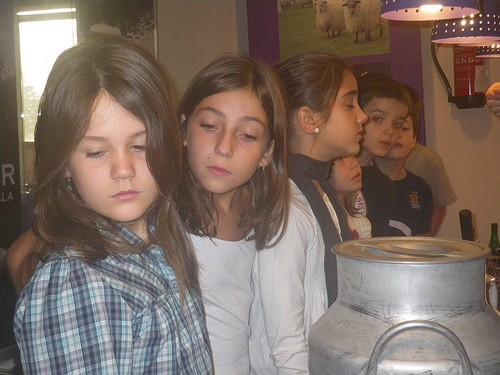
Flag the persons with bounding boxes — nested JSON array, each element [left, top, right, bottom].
[[483, 80, 500, 120], [345, 71, 398, 240], [391, 82, 460, 238], [373, 100, 436, 236], [153, 51, 286, 375], [244, 49, 368, 375], [13, 31, 219, 375], [325, 152, 373, 240]]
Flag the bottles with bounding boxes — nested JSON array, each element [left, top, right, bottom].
[[488, 223, 500, 256]]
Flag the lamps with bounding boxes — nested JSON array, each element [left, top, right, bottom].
[[475, 43, 500, 58], [431, 0, 500, 44], [379, 0, 482, 21]]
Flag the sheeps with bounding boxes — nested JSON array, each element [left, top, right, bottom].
[[341, 0, 382, 44], [313, 0, 347, 39]]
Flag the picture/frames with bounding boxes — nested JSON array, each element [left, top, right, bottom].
[[76, 0, 158, 64]]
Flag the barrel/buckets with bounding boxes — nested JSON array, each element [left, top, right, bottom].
[[308, 236, 500, 375]]
